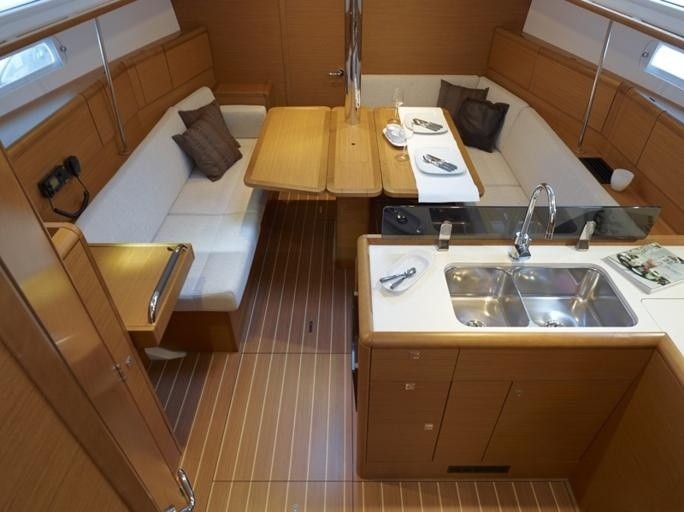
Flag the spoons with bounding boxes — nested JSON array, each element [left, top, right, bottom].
[[381, 267, 418, 290]]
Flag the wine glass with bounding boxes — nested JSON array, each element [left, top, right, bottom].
[[388, 87, 405, 124], [397, 120, 412, 162]]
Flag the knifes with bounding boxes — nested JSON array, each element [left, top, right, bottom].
[[413, 118, 443, 132], [423, 153, 457, 173]]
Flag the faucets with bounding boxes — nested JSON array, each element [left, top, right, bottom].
[[508, 182, 558, 257]]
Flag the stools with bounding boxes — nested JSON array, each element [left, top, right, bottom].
[[214, 81, 273, 110]]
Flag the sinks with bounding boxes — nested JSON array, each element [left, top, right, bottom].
[[443, 259, 528, 329], [516, 261, 640, 329]]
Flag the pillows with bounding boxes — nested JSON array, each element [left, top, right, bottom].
[[453, 97, 508, 153], [179, 96, 242, 149], [170, 110, 243, 184], [437, 81, 490, 118]]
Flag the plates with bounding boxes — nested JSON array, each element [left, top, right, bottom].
[[382, 127, 407, 147], [407, 120, 448, 134], [417, 154, 465, 175], [379, 255, 431, 293]]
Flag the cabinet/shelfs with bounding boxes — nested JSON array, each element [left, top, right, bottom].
[[12, 28, 217, 223]]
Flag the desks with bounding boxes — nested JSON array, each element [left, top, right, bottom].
[[84, 243, 194, 349], [244, 105, 484, 267]]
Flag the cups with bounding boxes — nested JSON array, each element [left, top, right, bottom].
[[385, 124, 407, 143], [610, 167, 635, 192]]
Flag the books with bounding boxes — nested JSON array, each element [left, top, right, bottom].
[[604, 241, 684, 294]]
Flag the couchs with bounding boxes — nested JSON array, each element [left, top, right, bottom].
[[71, 86, 268, 352], [359, 73, 645, 240]]
